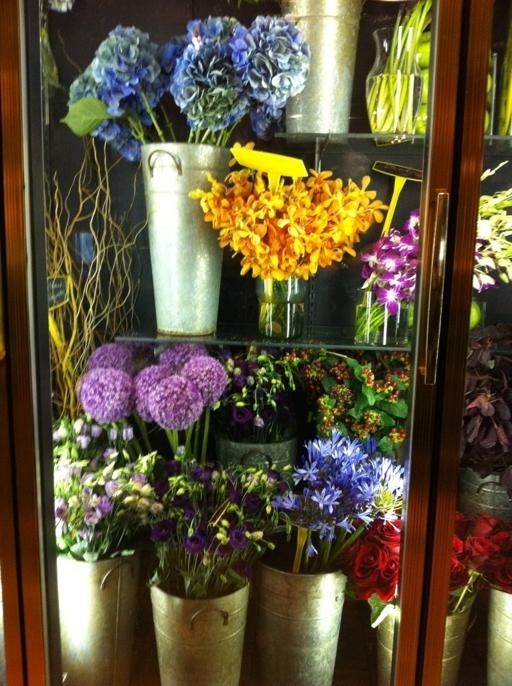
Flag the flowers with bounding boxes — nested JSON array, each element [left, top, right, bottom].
[[359, 186, 512, 315], [185, 139, 390, 283], [44, 336, 510, 628], [59, 11, 311, 164]]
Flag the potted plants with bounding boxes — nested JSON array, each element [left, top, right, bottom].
[[366, 0, 434, 135]]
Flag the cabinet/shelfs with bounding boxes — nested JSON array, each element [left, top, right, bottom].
[[0, 0, 511, 686]]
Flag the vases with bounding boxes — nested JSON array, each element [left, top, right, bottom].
[[252, 273, 316, 342], [254, 558, 349, 685], [43, 550, 144, 684], [139, 138, 235, 336], [484, 584, 511, 686], [372, 596, 474, 686], [147, 577, 254, 686], [278, 0, 362, 136], [353, 287, 404, 349]]
